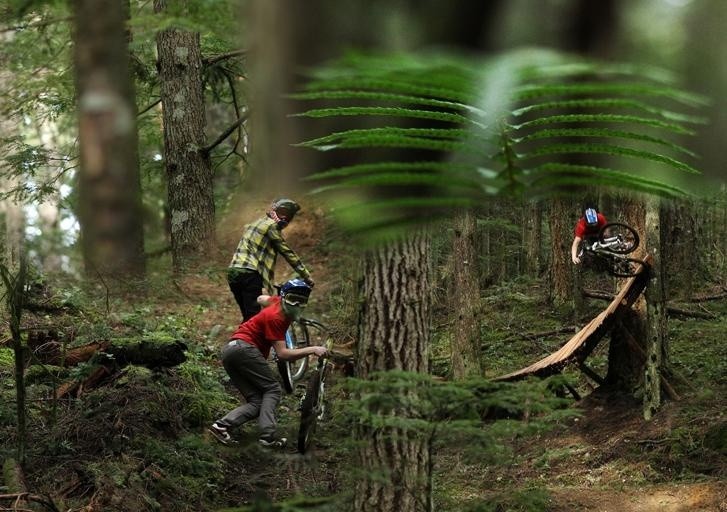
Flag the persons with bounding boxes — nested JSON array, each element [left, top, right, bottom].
[[571, 206, 609, 266], [208, 278, 327, 453], [228, 199, 315, 323]]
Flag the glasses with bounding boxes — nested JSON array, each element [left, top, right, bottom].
[[283, 292, 308, 308]]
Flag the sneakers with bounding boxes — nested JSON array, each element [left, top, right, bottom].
[[259, 438, 287, 450], [208, 422, 236, 448]]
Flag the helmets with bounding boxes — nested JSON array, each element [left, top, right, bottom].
[[272, 196, 300, 213], [280, 278, 311, 297], [584, 208, 598, 227]]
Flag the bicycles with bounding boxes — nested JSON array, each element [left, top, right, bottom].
[[298, 315, 356, 452], [577, 222, 649, 278], [271, 281, 310, 393]]
